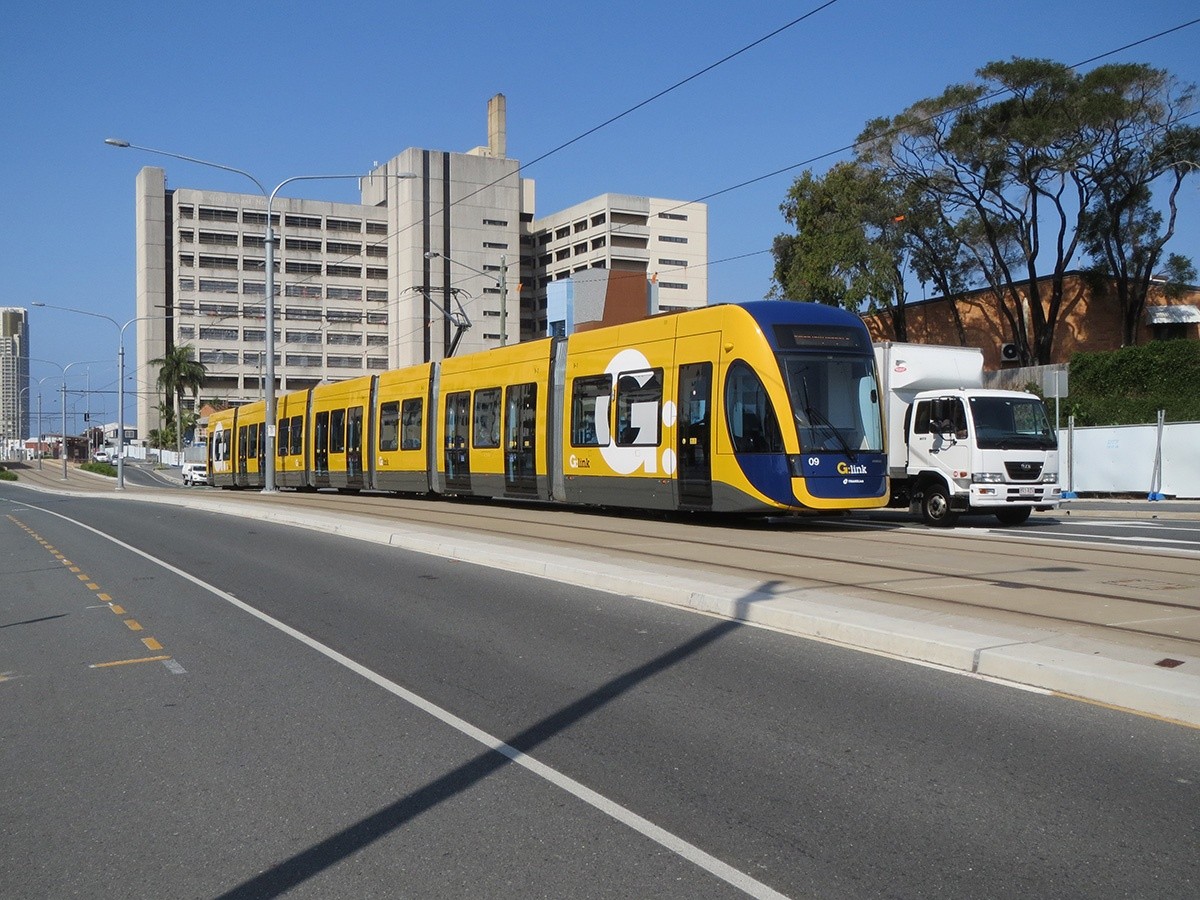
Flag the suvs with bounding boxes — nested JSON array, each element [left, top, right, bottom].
[[181, 463, 207, 486]]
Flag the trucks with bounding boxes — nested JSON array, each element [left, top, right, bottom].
[[825, 340, 1063, 529]]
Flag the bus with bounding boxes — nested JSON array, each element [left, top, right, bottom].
[[206, 302, 889, 522]]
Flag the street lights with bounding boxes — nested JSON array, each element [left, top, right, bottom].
[[425, 250, 506, 346], [30, 301, 209, 491], [0, 356, 120, 480], [103, 138, 417, 494]]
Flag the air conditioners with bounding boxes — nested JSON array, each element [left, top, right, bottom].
[[1001, 343, 1020, 361]]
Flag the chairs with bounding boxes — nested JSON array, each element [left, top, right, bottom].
[[382, 436, 465, 464], [278, 447, 287, 455]]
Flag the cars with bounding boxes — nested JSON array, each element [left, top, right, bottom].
[[94, 451, 108, 463], [111, 455, 118, 466]]
